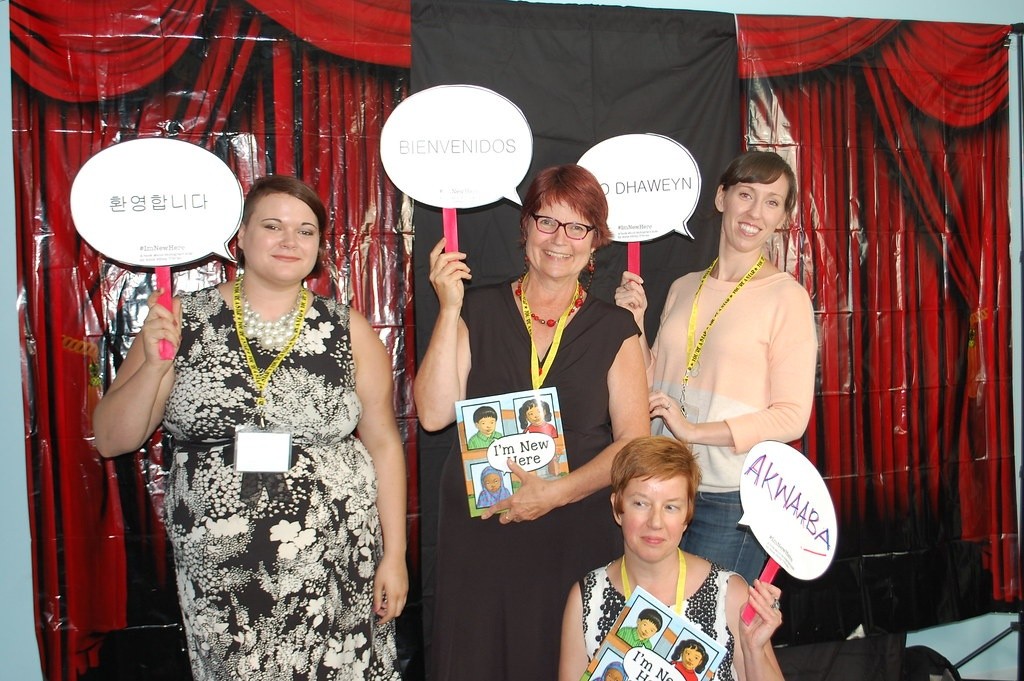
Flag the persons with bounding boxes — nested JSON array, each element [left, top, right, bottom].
[[412, 162, 648, 681], [558, 431, 789, 681], [93, 176, 411, 681], [614, 152, 817, 591]]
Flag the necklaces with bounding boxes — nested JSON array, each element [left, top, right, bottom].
[[240, 281, 302, 349], [515, 272, 584, 326]]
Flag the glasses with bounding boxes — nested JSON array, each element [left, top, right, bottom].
[[530, 211, 596, 240]]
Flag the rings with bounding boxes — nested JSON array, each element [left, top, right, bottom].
[[665, 401, 672, 410], [770, 598, 781, 611], [505, 512, 510, 521], [622, 282, 629, 291]]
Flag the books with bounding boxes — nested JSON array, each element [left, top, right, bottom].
[[454, 387, 571, 517], [578, 584, 729, 681]]
